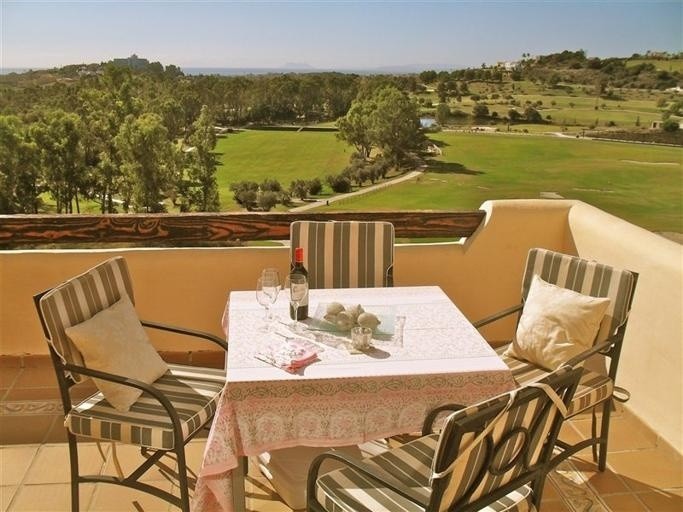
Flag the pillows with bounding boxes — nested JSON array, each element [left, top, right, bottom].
[[503, 275, 611, 385], [65, 295, 170, 412]]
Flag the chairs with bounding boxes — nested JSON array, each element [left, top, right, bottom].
[[32, 219, 639, 512]]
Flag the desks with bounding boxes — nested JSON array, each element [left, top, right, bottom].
[[190, 286, 517, 511]]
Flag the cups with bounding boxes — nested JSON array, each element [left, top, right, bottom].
[[350, 327, 372, 350]]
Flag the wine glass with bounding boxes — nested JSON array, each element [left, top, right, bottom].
[[255, 267, 309, 332]]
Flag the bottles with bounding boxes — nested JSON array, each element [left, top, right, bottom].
[[288, 247, 308, 319]]
